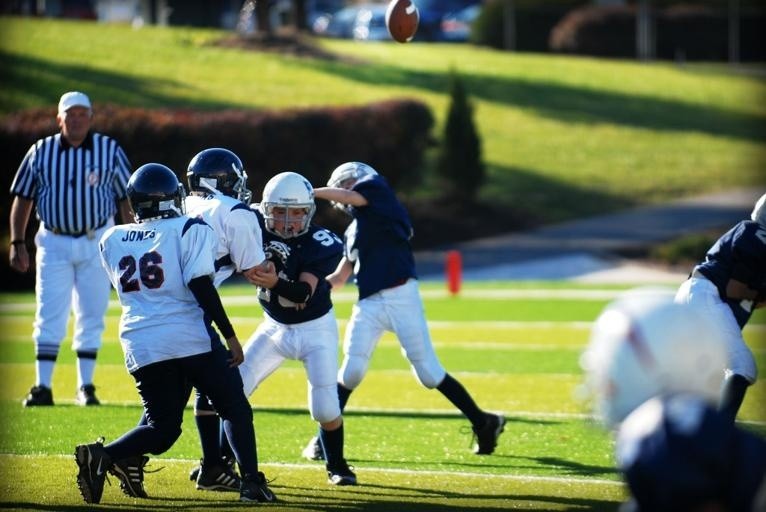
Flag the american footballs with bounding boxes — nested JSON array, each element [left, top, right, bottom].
[[386, 0, 419, 42]]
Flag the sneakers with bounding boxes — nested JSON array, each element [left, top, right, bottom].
[[303, 436, 325, 461], [77, 384, 99, 406], [109, 451, 151, 500], [75, 442, 108, 505], [192, 455, 277, 503], [22, 383, 55, 406], [469, 413, 508, 456], [327, 457, 358, 486]]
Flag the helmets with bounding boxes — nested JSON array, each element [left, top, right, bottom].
[[589, 285, 762, 510], [186, 147, 248, 201], [261, 172, 316, 237], [327, 160, 378, 192], [125, 162, 184, 218]]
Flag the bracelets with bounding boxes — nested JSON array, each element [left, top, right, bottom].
[[9, 238, 27, 247]]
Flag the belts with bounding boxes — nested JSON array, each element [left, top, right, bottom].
[[43, 220, 107, 239]]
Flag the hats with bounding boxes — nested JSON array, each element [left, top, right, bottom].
[[58, 90, 91, 112]]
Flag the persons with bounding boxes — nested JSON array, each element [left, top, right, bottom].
[[74, 160, 277, 503], [189, 170, 358, 488], [585, 283, 765, 512], [7, 91, 135, 406], [686, 191, 766, 424], [111, 148, 286, 499], [305, 161, 507, 462]]
